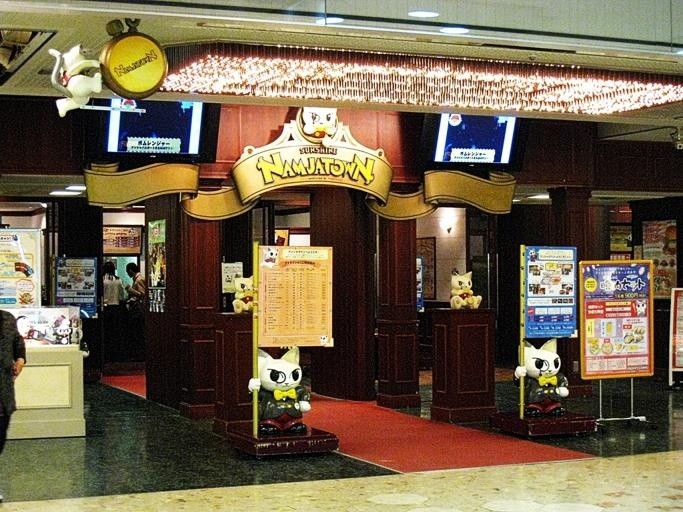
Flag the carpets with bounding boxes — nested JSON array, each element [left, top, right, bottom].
[[97, 370, 599, 477]]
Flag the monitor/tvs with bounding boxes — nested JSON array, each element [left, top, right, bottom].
[[84, 99, 221, 164], [414, 113, 532, 172]]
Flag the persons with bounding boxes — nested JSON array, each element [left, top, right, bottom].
[[0, 309, 28, 502], [123, 262, 146, 362], [100, 262, 125, 362]]
